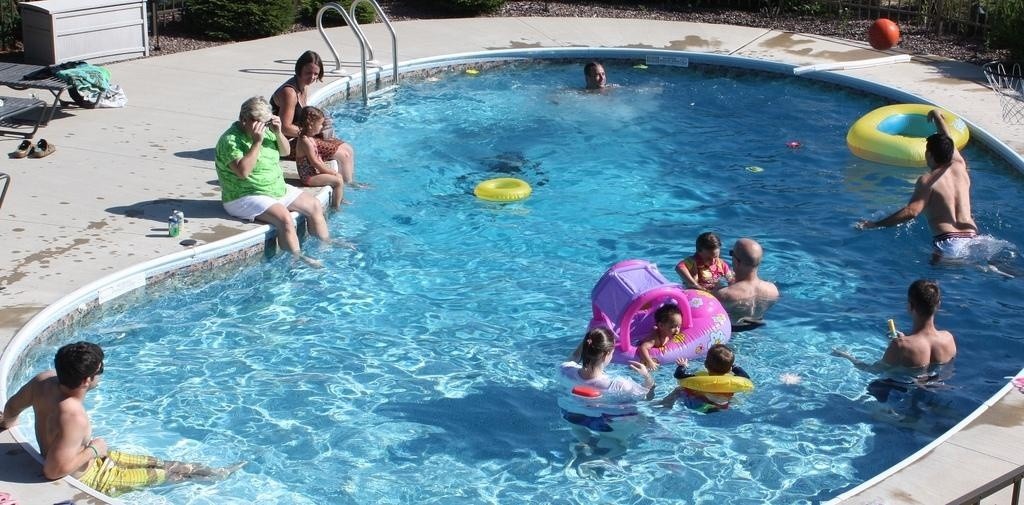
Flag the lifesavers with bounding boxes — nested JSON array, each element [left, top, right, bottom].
[[586, 261, 732, 365], [473, 178, 531, 202], [846, 102, 969, 168], [677, 369, 753, 393]]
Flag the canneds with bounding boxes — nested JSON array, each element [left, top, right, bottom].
[[175, 210, 185, 232], [168, 216, 179, 237]]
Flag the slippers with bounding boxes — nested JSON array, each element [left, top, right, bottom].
[[15, 139, 54, 158]]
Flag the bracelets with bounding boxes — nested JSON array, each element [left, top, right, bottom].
[[88, 444, 99, 458]]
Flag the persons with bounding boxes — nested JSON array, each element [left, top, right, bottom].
[[558, 326, 654, 416], [657, 343, 750, 414], [830, 277, 957, 375], [270, 51, 368, 190], [0, 341, 248, 498], [585, 60, 607, 91], [295, 105, 352, 213], [639, 304, 683, 372], [712, 239, 779, 332], [214, 96, 329, 269], [857, 110, 1024, 279], [675, 232, 736, 293]]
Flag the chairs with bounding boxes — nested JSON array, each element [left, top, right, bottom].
[[0, 94, 47, 138], [0, 59, 87, 122], [0, 171, 11, 207]]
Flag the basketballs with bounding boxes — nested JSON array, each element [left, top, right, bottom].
[[869, 18, 899, 50]]
